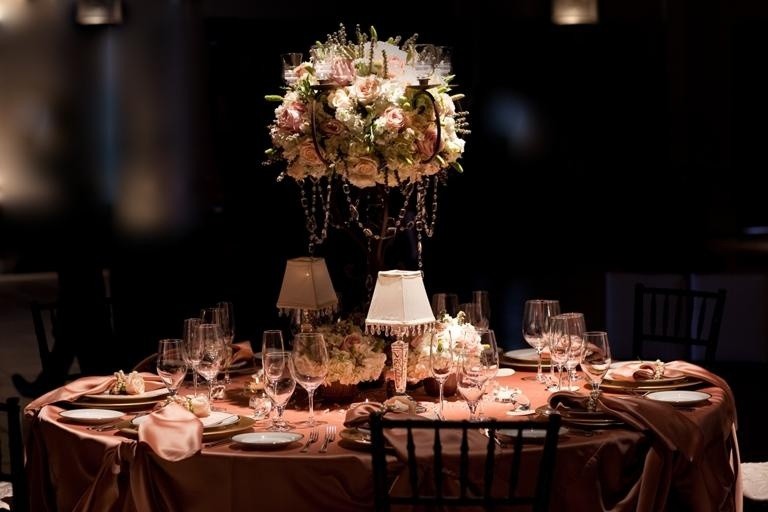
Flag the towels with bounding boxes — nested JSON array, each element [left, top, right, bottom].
[[22, 372, 165, 419], [138, 401, 204, 463], [547, 390, 699, 451], [611, 359, 738, 431]]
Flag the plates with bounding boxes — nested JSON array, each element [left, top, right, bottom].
[[338, 427, 372, 448], [131, 407, 240, 430], [115, 415, 256, 441], [66, 399, 168, 409], [576, 422, 625, 426], [503, 348, 558, 363], [231, 431, 304, 449], [644, 390, 712, 405], [506, 408, 536, 416], [499, 361, 557, 370], [603, 368, 686, 383], [563, 416, 617, 423], [599, 379, 704, 393], [83, 387, 171, 400]]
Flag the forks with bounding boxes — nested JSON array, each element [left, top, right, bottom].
[[300, 431, 319, 453], [319, 425, 336, 452]]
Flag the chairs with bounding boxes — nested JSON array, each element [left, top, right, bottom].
[[30, 298, 123, 383], [369, 414, 562, 511], [632, 282, 680, 360]]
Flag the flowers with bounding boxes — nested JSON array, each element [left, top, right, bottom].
[[406, 309, 489, 385], [261, 24, 471, 246], [309, 320, 388, 386]]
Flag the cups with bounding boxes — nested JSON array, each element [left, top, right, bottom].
[[429, 330, 453, 420], [184, 318, 205, 397], [201, 301, 232, 384], [473, 291, 489, 329], [432, 294, 458, 316], [289, 333, 329, 427], [557, 313, 586, 382], [465, 330, 499, 420], [548, 317, 570, 391], [262, 330, 285, 415], [264, 352, 296, 432], [456, 353, 488, 421], [460, 302, 473, 323], [193, 324, 226, 405], [580, 332, 612, 393], [156, 339, 189, 396], [521, 299, 561, 348]]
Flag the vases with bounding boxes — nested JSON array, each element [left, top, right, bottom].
[[316, 382, 356, 408], [419, 366, 459, 398]]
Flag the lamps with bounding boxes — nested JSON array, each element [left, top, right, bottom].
[[363, 270, 436, 414], [277, 255, 339, 333]]
